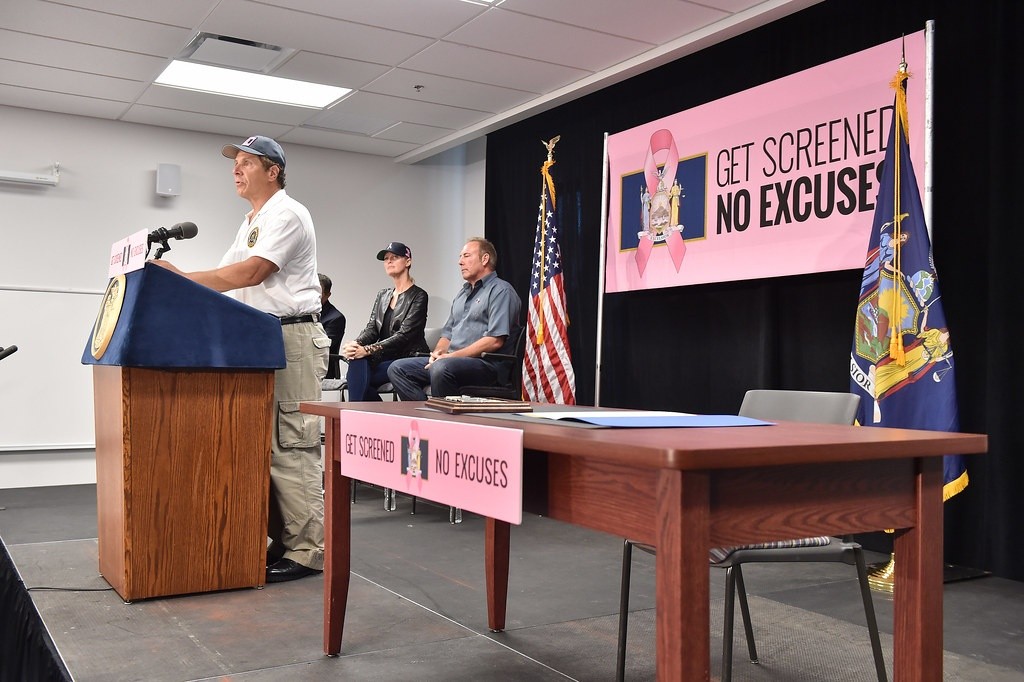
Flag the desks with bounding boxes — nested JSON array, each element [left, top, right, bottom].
[[299, 396, 986, 682]]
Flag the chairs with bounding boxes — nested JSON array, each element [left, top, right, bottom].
[[410, 324, 527, 526], [342, 327, 444, 512], [617, 388, 888, 682]]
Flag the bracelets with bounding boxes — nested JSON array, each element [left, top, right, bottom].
[[364, 345, 374, 354], [355, 340, 365, 346]]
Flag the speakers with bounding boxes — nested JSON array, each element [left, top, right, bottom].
[[156, 162, 182, 196]]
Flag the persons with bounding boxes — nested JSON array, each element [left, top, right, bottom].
[[522, 161, 576, 405], [150, 136, 332, 581], [318, 273, 346, 379], [387, 239, 522, 402], [342, 241, 431, 402]]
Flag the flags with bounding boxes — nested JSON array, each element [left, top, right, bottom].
[[850, 71, 969, 534]]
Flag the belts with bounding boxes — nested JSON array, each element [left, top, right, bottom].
[[280, 314, 320, 325]]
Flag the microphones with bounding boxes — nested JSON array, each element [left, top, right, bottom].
[[148, 221, 198, 243]]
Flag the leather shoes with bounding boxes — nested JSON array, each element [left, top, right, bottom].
[[266, 558, 323, 582], [266, 541, 287, 567]]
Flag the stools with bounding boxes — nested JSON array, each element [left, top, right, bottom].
[[318, 354, 354, 403]]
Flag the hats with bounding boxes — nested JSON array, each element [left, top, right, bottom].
[[377, 242, 411, 261], [222, 135, 286, 168]]
